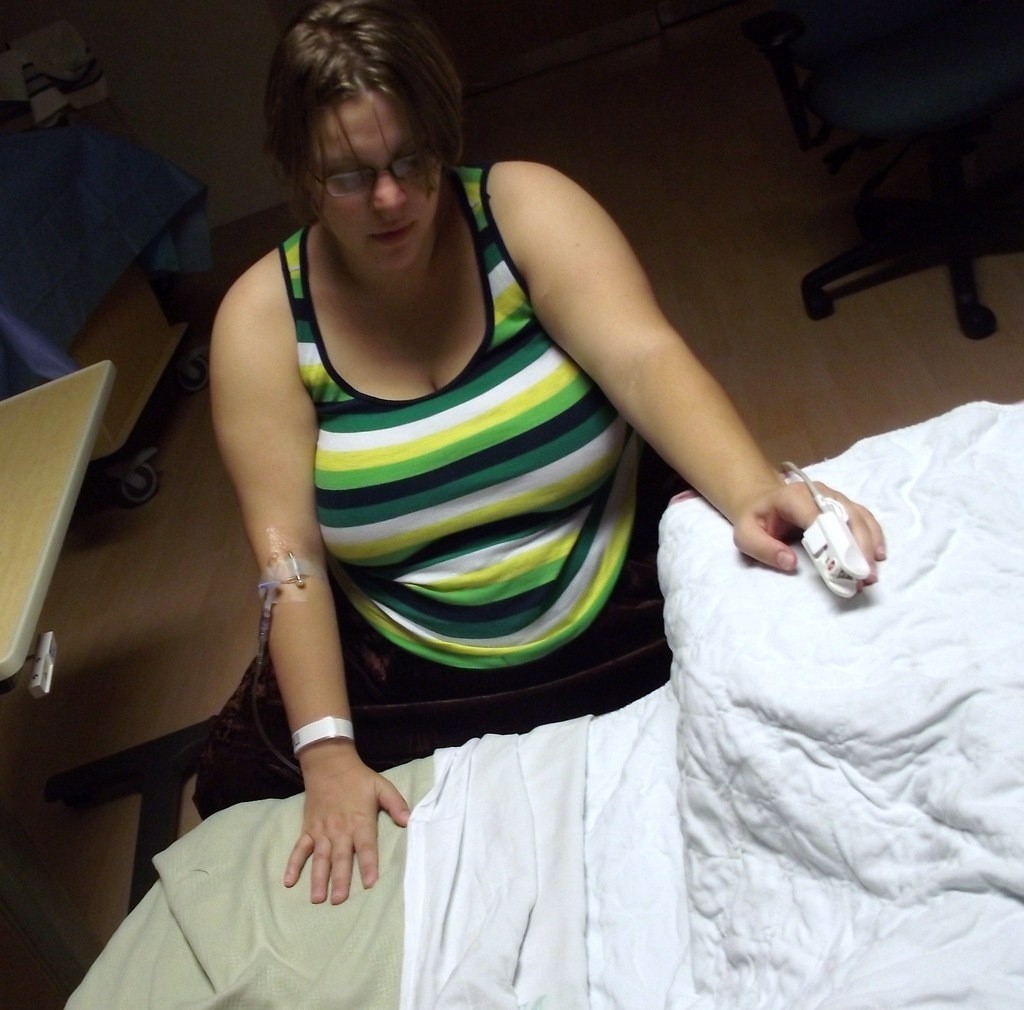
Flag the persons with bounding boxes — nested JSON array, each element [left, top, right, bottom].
[[194, 0, 885, 903]]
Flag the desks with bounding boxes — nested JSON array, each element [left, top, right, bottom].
[[0, 359, 120, 679]]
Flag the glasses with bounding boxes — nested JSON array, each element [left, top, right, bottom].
[[305, 140, 441, 196]]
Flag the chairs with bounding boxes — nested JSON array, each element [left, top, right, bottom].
[[755, 0, 1024, 340]]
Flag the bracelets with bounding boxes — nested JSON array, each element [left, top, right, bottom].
[[293, 715, 355, 759]]
